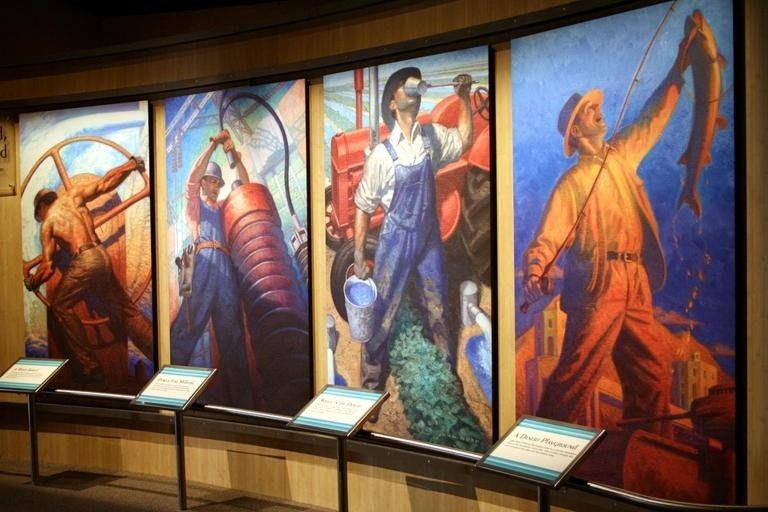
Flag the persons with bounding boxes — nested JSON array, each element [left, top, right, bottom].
[[24, 156, 155, 387], [521, 27, 698, 479], [170, 129, 251, 416], [351, 66, 474, 428]]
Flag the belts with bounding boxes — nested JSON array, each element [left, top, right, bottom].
[[71, 239, 101, 260], [193, 241, 232, 256], [606, 250, 643, 265]]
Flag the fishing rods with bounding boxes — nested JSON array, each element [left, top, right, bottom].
[[521, 1, 678, 314]]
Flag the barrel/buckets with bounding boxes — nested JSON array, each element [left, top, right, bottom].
[[343, 263, 379, 346]]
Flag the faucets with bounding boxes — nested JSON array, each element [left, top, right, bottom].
[[459, 280, 481, 331]]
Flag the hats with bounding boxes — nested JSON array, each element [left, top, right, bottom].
[[381, 67, 422, 130], [201, 161, 225, 187], [33, 188, 57, 222], [557, 88, 603, 157]]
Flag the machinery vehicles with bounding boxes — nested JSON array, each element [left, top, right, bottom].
[[326, 60, 489, 350]]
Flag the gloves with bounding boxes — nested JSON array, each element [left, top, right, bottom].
[[130, 155, 146, 172], [23, 274, 35, 292]]
